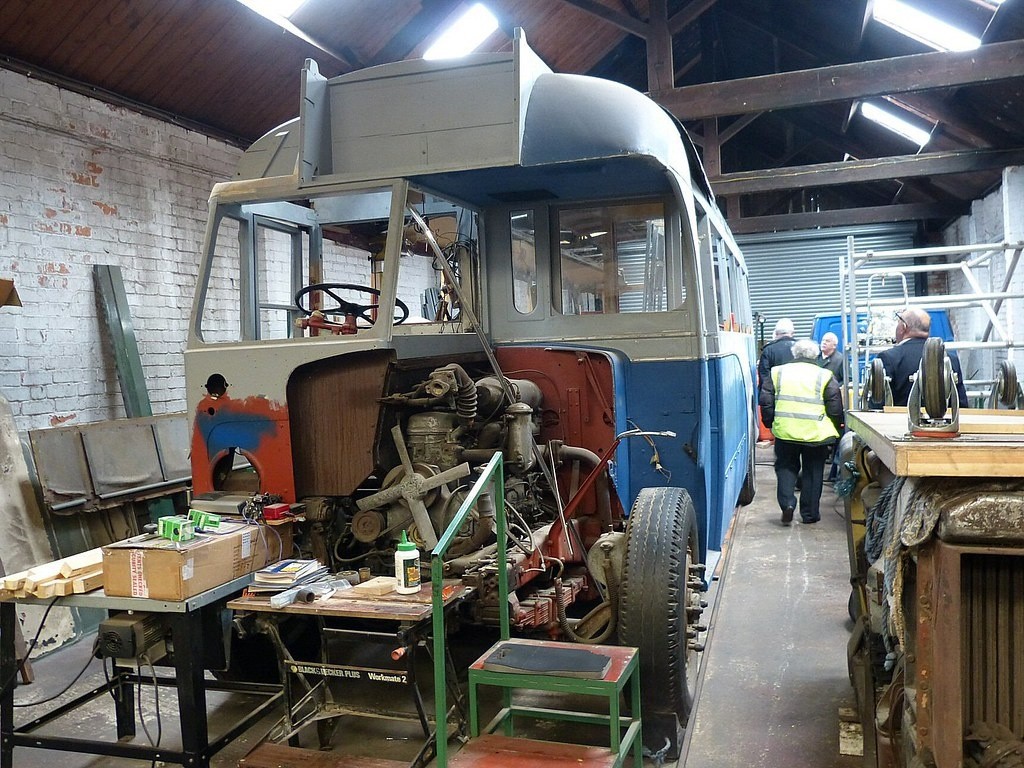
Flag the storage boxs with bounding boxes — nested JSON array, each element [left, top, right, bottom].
[[102, 522, 268, 603]]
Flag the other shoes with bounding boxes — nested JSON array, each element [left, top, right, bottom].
[[782, 506, 794, 523], [804, 515, 820, 523]]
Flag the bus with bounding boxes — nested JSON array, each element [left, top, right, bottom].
[[181, 26, 759, 717]]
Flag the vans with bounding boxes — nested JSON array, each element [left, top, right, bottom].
[[812, 309, 968, 408]]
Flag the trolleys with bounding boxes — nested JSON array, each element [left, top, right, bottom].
[[844, 336, 1023, 478]]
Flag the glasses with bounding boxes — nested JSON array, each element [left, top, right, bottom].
[[893, 312, 909, 327]]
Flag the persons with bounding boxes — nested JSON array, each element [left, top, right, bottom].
[[814, 332, 844, 388], [757, 318, 798, 407], [758, 338, 845, 525], [869, 307, 970, 408]]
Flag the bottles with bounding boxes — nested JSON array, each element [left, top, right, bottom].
[[394, 529, 421, 594]]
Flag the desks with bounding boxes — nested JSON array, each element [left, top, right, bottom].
[[0, 569, 463, 768]]
